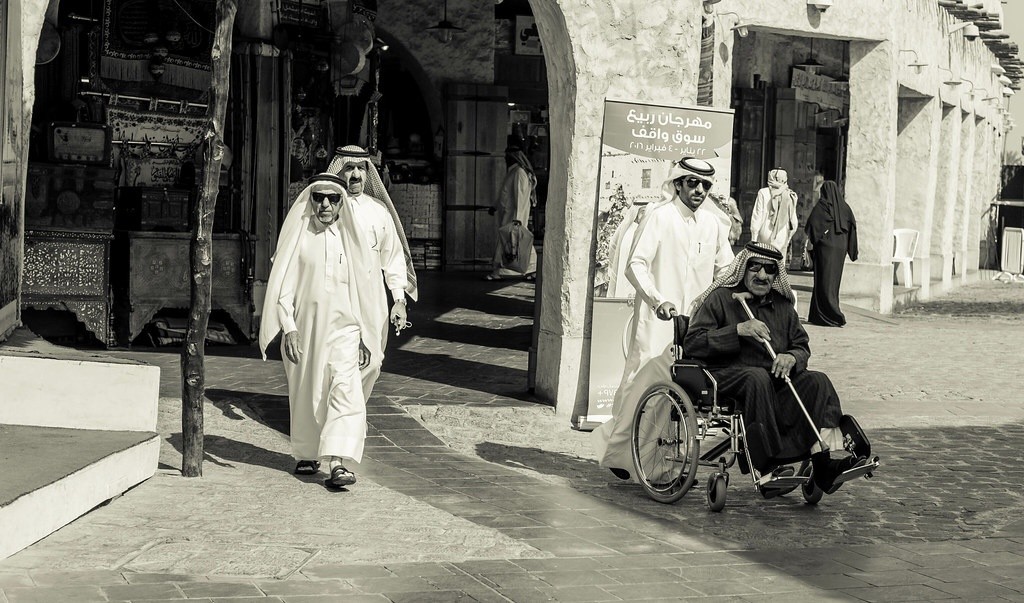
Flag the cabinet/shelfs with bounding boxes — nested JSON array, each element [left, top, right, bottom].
[[24, 163, 253, 350]]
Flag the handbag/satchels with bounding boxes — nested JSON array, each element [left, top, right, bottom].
[[493, 223, 534, 272], [800, 235, 813, 271]]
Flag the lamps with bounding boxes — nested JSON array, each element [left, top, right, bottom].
[[716, 10, 752, 38], [426, 0, 468, 42], [958, 76, 977, 101], [991, 95, 1016, 131], [796, 38, 824, 75], [827, 40, 849, 91], [899, 49, 928, 75], [976, 87, 995, 105], [805, 101, 828, 121], [938, 64, 963, 91], [828, 107, 848, 127]]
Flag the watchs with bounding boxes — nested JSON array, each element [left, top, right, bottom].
[[395, 298, 407, 306]]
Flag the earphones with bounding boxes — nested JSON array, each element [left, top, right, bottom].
[[396, 328, 400, 337]]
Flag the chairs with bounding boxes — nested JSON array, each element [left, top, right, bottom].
[[893, 228, 920, 289]]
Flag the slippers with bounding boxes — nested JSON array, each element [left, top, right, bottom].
[[295, 460, 317, 472], [524, 272, 536, 280], [486, 275, 502, 282], [325, 465, 356, 487]]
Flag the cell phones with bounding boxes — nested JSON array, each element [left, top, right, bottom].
[[395, 316, 399, 331]]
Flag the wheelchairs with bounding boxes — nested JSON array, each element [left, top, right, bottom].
[[630, 308, 881, 513]]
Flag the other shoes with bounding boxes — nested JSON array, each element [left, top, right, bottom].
[[811, 451, 865, 494], [609, 468, 630, 481], [761, 467, 794, 499], [673, 476, 698, 486]]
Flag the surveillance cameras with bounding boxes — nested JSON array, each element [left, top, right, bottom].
[[964, 25, 980, 41]]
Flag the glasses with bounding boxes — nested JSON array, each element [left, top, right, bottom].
[[684, 177, 712, 190], [312, 192, 342, 203], [747, 261, 778, 274]]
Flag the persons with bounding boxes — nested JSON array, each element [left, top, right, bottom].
[[592, 156, 735, 487], [259, 172, 385, 485], [487, 146, 537, 281], [685, 240, 844, 477], [326, 145, 417, 403], [606, 198, 649, 297], [803, 180, 858, 327], [750, 169, 798, 264]]
